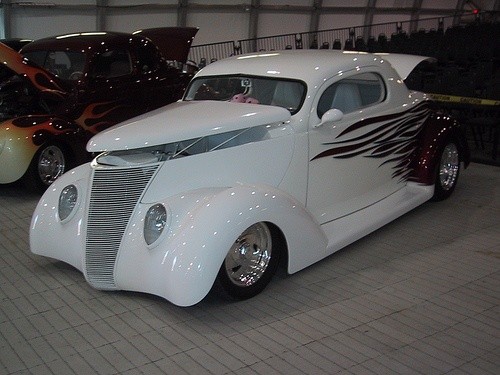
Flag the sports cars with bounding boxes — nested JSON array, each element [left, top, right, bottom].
[[0, 25, 202, 198], [27, 46, 472, 309]]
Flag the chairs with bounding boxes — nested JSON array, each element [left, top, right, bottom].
[[332, 84, 361, 115], [270, 81, 304, 109]]
